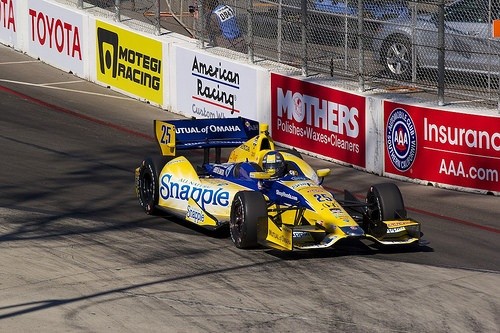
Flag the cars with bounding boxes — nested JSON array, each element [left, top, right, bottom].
[[371, 1, 499, 82]]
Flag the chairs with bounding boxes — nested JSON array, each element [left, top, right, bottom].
[[233, 160, 263, 179]]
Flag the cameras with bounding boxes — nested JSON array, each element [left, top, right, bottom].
[[189, 5, 198, 12]]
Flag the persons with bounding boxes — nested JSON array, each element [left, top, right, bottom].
[[201, 0, 248, 53], [262, 151, 286, 180]]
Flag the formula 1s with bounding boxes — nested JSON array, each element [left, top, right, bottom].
[[135, 115, 432, 254]]
